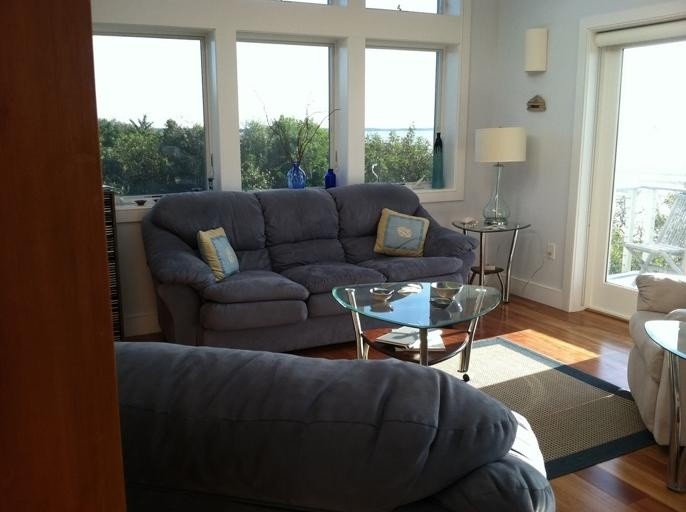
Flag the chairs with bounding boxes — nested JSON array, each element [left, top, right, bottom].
[[112, 341, 556, 512], [628, 272, 686, 445]]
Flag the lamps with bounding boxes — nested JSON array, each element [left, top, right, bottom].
[[473, 123, 527, 224], [523, 26, 548, 71]]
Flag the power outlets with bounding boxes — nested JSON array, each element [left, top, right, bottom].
[[548, 242, 555, 259]]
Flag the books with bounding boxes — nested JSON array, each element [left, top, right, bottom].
[[391, 328, 446, 353], [375, 325, 420, 349]]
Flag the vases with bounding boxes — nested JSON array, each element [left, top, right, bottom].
[[288, 161, 307, 188]]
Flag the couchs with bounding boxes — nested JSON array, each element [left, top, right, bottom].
[[140, 181, 479, 353]]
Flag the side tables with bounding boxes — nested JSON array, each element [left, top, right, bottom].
[[450, 216, 531, 303], [644, 320, 686, 495]]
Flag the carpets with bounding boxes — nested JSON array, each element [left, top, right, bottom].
[[429, 336, 653, 480]]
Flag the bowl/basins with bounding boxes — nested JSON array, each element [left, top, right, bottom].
[[448, 299, 464, 313], [369, 301, 394, 313], [430, 280, 464, 299], [428, 296, 453, 309], [370, 287, 395, 301], [428, 310, 452, 321], [134, 200, 147, 206]]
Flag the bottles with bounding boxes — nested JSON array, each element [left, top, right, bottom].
[[325, 168, 336, 189], [432, 132, 444, 190]]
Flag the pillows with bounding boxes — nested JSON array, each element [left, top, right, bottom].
[[195, 225, 241, 280], [375, 207, 429, 257]]
